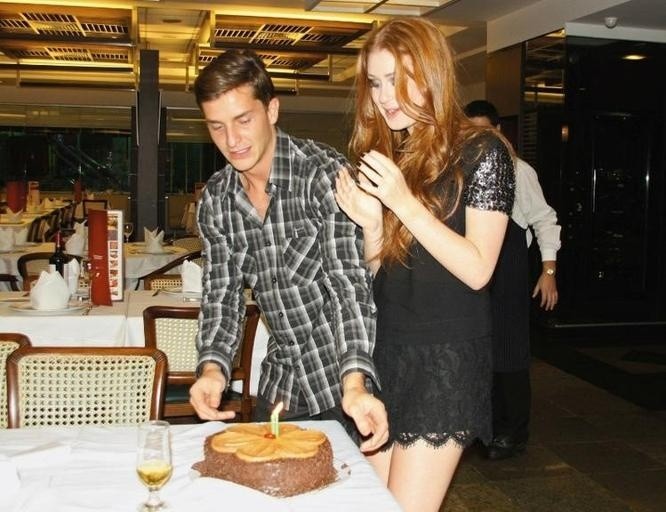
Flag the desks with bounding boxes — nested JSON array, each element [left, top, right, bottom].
[[0, 420, 406, 512]]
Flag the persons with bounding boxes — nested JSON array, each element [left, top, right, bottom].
[[331, 19, 516, 512], [463, 100, 561, 460], [186, 50, 389, 453]]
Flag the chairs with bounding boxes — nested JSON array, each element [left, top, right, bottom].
[[1, 181, 262, 428]]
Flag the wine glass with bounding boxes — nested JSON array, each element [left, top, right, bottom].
[[123, 222, 135, 250], [135, 419, 173, 512]]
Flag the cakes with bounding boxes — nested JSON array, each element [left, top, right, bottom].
[[191, 423, 338, 498]]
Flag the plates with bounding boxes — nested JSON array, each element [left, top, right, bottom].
[[0, 234, 180, 256], [1, 288, 203, 318]]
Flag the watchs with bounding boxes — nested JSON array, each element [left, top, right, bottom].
[[542, 267, 556, 276]]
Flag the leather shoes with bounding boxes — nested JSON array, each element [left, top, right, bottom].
[[470, 429, 527, 460]]
